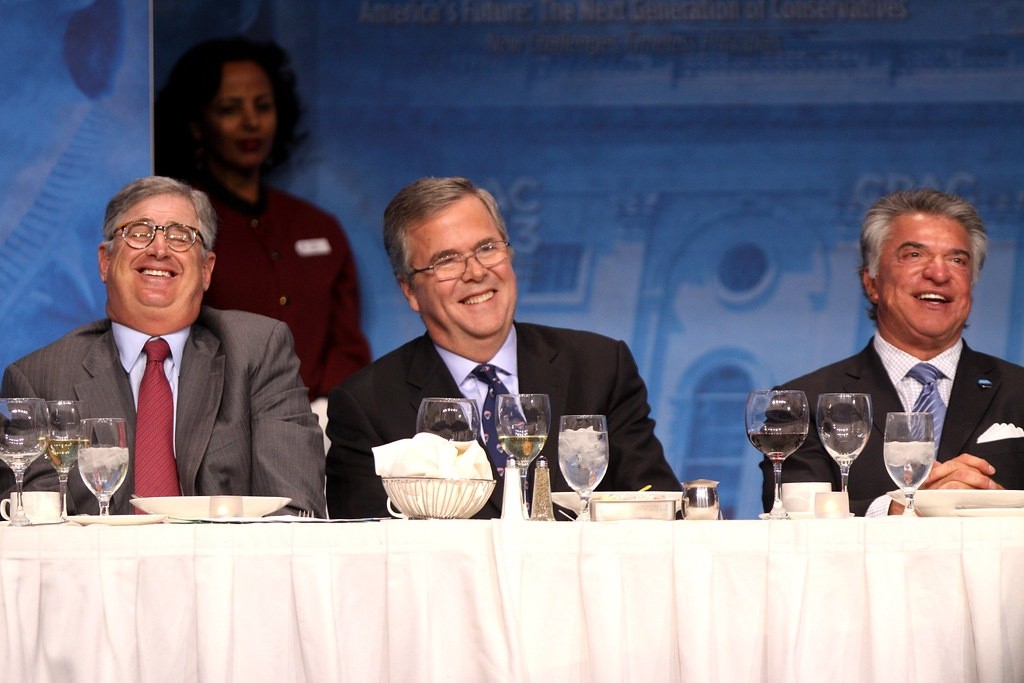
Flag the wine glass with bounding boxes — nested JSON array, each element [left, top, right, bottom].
[[816, 393, 873, 518], [495, 393, 550, 519], [0, 398, 50, 526], [41, 400, 91, 516], [78, 417, 129, 515], [558, 413, 609, 522], [745, 389, 809, 520], [883, 411, 935, 517]]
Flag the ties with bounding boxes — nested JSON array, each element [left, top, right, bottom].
[[907, 361, 946, 465], [473, 364, 533, 515], [135, 340, 181, 514]]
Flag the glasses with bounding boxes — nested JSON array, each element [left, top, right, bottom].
[[108, 219, 207, 253], [406, 240, 512, 281]]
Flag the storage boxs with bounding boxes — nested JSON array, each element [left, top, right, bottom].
[[589, 492, 680, 520]]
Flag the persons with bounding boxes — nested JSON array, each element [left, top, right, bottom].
[[155, 36, 372, 403], [325, 178, 685, 522], [0, 177, 325, 524], [758, 187, 1024, 517]]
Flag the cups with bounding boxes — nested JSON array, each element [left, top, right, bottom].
[[416, 397, 478, 444], [0, 491, 60, 521], [778, 481, 849, 515], [680, 479, 721, 521], [386, 497, 409, 520]]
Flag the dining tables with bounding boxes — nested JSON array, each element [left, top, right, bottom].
[[0, 517, 1024, 683]]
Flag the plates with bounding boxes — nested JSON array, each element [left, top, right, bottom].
[[128, 497, 291, 520], [887, 491, 1024, 516], [549, 491, 686, 517], [62, 515, 167, 526], [760, 512, 855, 519]]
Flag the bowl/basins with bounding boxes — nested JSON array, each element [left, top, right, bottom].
[[382, 477, 496, 519]]
